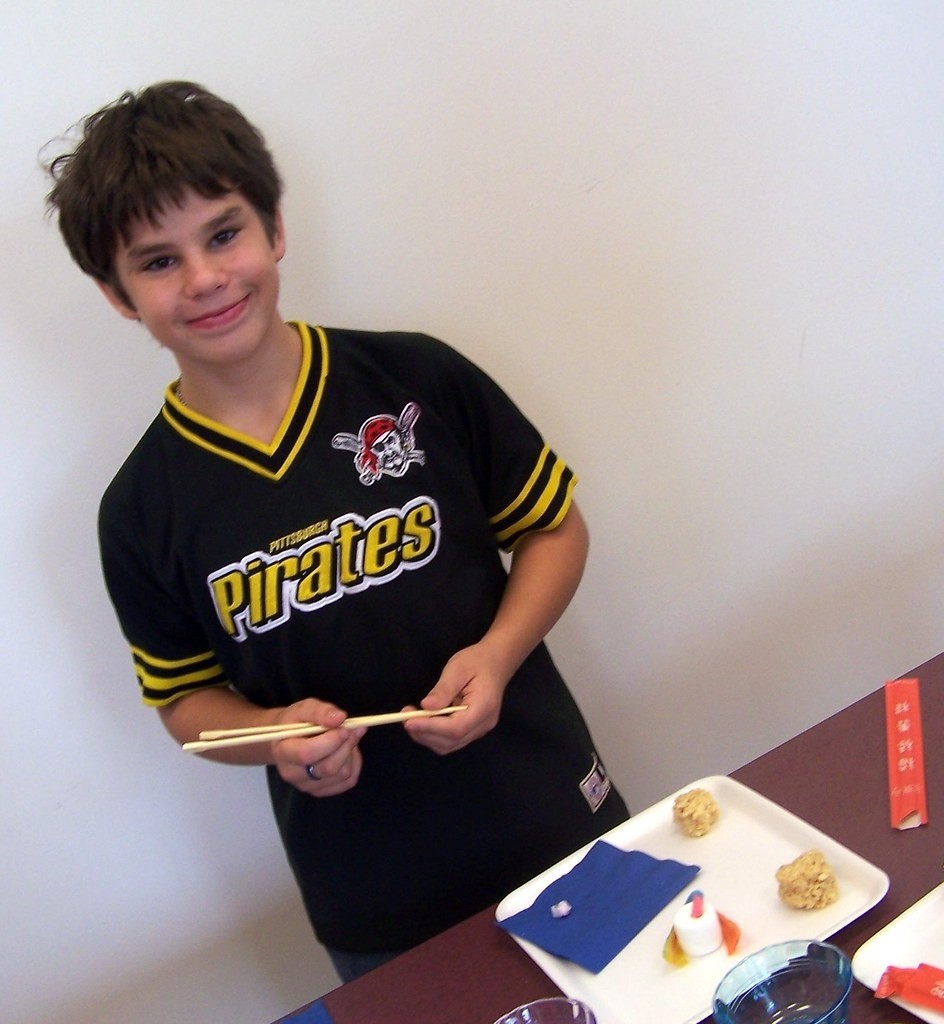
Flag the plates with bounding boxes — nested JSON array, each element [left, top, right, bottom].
[[493, 774, 893, 1024], [852, 881, 944, 1024]]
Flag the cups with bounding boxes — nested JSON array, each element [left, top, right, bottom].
[[492, 997, 602, 1024], [712, 939, 854, 1024]]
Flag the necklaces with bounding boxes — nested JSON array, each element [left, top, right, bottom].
[[176, 324, 295, 405]]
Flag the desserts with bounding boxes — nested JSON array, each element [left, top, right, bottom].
[[673, 790, 719, 836], [775, 852, 840, 910], [673, 902, 723, 956]]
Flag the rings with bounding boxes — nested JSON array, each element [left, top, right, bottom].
[[306, 766, 321, 781]]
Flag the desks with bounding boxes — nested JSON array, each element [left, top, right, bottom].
[[272, 651, 944, 1024]]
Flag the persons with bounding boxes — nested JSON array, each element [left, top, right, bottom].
[[43, 81, 632, 983]]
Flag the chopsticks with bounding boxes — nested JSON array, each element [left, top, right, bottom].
[[180, 704, 468, 756]]
